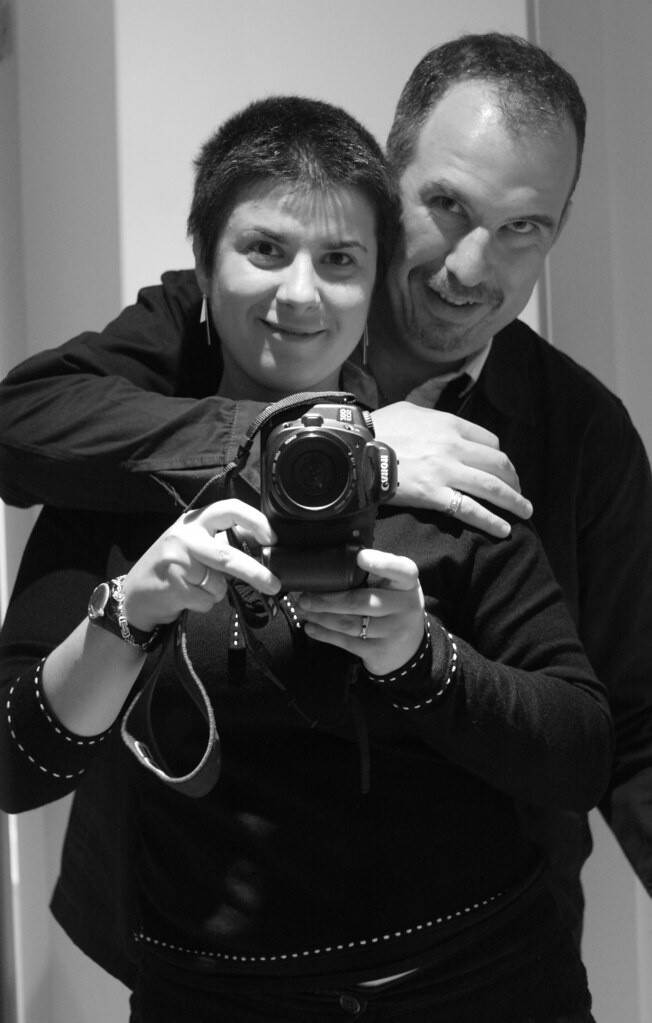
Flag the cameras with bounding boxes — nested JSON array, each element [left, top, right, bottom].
[[251, 401, 400, 595]]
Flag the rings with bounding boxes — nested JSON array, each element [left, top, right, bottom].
[[358, 615, 370, 639]]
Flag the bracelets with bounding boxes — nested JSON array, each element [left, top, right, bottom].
[[110, 574, 158, 650]]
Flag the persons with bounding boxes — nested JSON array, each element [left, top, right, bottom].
[[0, 31, 652, 894], [0, 94, 610, 1023]]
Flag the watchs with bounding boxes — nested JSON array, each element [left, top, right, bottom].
[[87, 582, 158, 646]]
